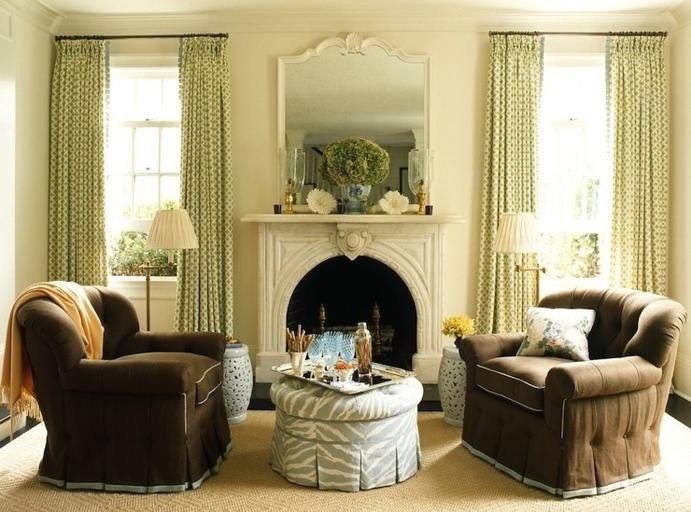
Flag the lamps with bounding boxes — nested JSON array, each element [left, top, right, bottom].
[[146, 208, 200, 268], [494, 211, 551, 272]]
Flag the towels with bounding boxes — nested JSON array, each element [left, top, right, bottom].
[[1, 282, 106, 423]]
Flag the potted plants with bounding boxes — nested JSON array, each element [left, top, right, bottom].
[[319, 137, 390, 215], [322, 156, 343, 206]]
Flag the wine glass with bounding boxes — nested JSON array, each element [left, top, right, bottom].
[[306, 331, 355, 370]]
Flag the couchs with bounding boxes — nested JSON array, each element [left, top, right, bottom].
[[458, 286, 687, 498], [20, 285, 231, 493]]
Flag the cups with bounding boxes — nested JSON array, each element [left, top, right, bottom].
[[274, 204, 281, 214], [425, 205, 433, 215], [335, 367, 353, 381], [288, 351, 307, 374], [337, 203, 345, 214]]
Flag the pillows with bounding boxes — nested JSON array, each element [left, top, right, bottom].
[[516, 306, 595, 360]]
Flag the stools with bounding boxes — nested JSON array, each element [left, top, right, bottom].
[[268, 361, 424, 490], [438, 346, 467, 426], [210, 344, 253, 425]]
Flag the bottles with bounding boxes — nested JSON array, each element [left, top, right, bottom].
[[355, 321, 373, 376]]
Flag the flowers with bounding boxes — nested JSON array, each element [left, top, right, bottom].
[[442, 314, 476, 337]]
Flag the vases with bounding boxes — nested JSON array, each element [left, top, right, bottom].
[[454, 337, 462, 348]]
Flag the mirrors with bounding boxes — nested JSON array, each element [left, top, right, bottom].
[[277, 33, 432, 212]]
